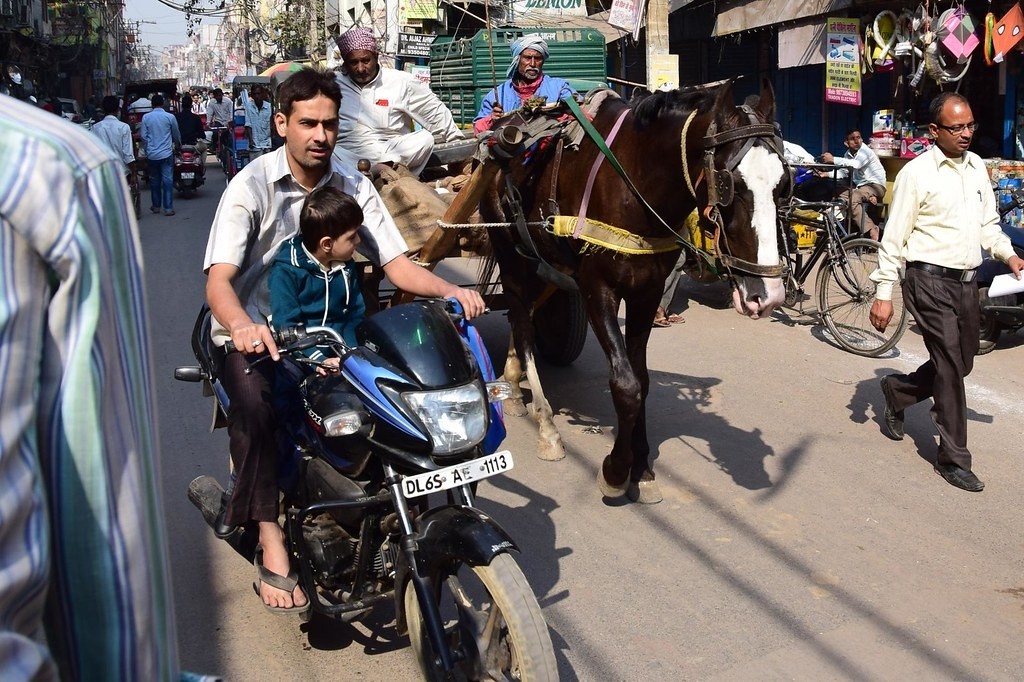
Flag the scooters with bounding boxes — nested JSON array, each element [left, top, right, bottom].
[[974, 188, 1024, 356], [173, 145, 207, 199]]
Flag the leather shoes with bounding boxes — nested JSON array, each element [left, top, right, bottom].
[[881, 375, 905, 439], [935, 461, 985, 491], [213, 492, 239, 540]]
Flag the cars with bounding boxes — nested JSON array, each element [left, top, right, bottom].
[[58, 97, 86, 125]]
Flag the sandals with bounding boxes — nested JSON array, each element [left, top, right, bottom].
[[666, 314, 685, 323], [653, 317, 671, 326]]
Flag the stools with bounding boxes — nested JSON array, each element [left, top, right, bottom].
[[855, 199, 890, 253]]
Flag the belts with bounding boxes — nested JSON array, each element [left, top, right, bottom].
[[906, 261, 979, 282]]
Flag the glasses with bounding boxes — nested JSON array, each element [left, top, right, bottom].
[[937, 122, 978, 136]]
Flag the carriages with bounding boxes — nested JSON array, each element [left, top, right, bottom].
[[356, 73, 796, 505]]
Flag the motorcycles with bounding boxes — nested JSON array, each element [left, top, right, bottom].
[[173, 295, 560, 681]]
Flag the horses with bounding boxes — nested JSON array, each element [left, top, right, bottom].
[[466, 71, 789, 504]]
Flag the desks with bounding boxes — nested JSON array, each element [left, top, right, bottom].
[[235, 118, 249, 149]]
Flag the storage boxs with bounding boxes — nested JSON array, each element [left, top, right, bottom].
[[900, 137, 934, 160]]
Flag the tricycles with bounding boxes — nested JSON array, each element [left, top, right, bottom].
[[119, 69, 296, 190]]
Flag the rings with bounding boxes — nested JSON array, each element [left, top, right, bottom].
[[252, 341, 263, 347]]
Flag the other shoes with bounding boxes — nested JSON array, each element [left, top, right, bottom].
[[165, 211, 175, 216], [150, 208, 160, 213]]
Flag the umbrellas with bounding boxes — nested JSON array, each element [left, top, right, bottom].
[[259, 63, 303, 76]]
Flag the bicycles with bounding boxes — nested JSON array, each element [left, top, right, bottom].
[[680, 161, 911, 357]]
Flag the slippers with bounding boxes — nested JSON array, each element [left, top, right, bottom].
[[254, 553, 310, 614]]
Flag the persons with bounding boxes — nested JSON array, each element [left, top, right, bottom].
[[473, 35, 584, 137], [813, 128, 886, 248], [266, 186, 367, 424], [331, 28, 466, 182], [87, 82, 273, 213], [868, 92, 1024, 491], [201, 68, 485, 616], [0, 96, 227, 682]]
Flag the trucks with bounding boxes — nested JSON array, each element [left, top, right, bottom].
[[429, 27, 608, 130]]
